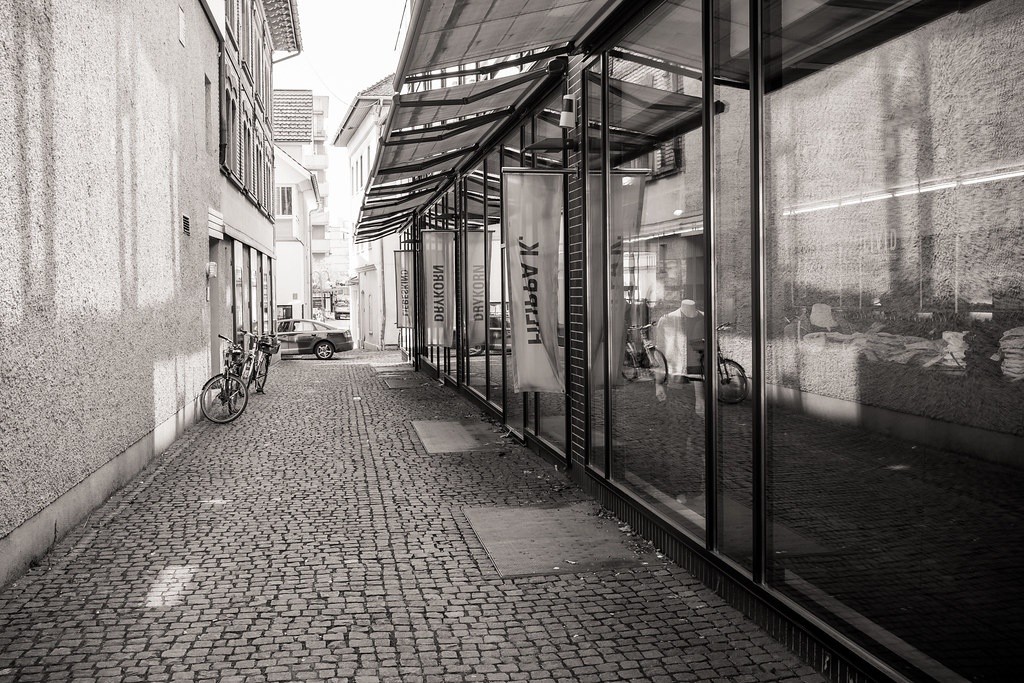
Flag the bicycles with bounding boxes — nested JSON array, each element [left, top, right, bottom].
[[237, 328, 272, 398], [621, 320, 668, 384], [199, 334, 253, 424], [257, 331, 281, 365], [689, 322, 750, 405]]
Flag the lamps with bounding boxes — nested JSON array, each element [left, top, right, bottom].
[[433, 214, 455, 219], [521, 138, 564, 153], [401, 239, 419, 243]]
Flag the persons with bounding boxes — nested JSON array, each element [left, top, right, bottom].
[[313, 307, 329, 323], [650, 299, 707, 507]]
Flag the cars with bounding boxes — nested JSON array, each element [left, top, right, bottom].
[[465, 307, 513, 358], [275, 318, 354, 360], [335, 302, 351, 320]]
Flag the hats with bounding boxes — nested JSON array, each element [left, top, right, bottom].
[[810, 303, 839, 328]]
